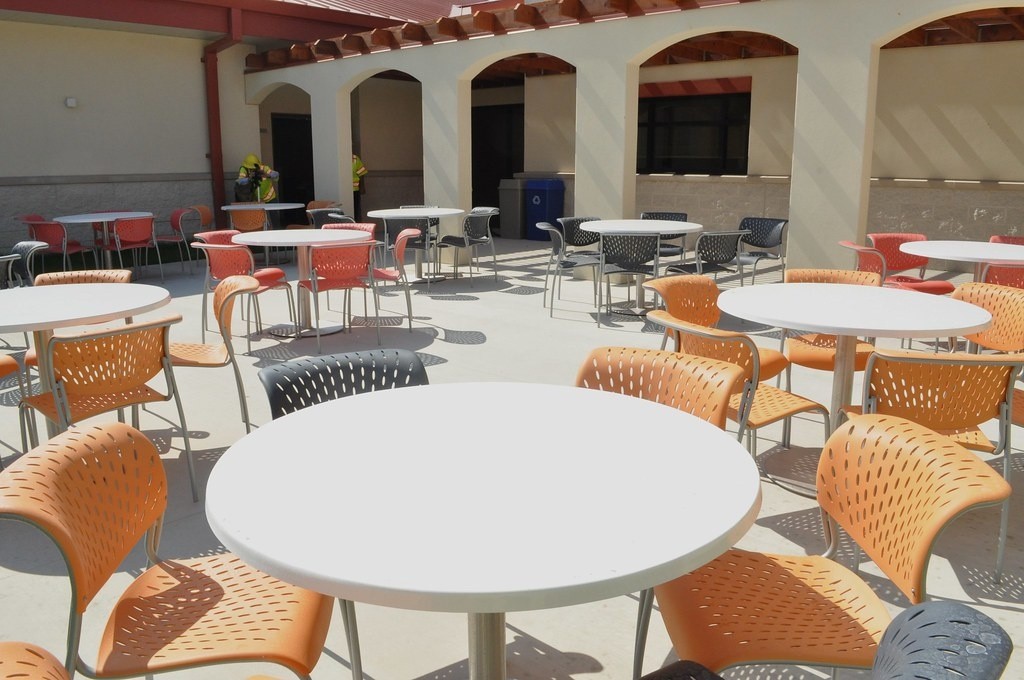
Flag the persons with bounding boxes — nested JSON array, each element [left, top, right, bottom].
[[236, 154, 281, 231], [352, 154, 367, 223]]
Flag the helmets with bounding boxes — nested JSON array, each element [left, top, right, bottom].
[[241, 154, 259, 169]]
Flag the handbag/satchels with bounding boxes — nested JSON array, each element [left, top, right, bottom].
[[234, 166, 254, 202]]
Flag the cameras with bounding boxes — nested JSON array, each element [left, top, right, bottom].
[[253, 164, 264, 182]]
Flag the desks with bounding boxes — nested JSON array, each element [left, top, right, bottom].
[[231, 229, 372, 338], [899, 240, 1024, 283], [203, 382, 762, 680], [0, 284, 171, 440], [53, 212, 154, 270], [579, 219, 704, 317], [715, 283, 991, 444], [220, 203, 305, 270], [367, 208, 466, 283]]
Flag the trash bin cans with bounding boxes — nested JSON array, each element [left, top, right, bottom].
[[524, 178, 565, 242], [498, 178, 525, 240]]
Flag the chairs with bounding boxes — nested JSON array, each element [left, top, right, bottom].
[[0, 201, 1024, 680]]
